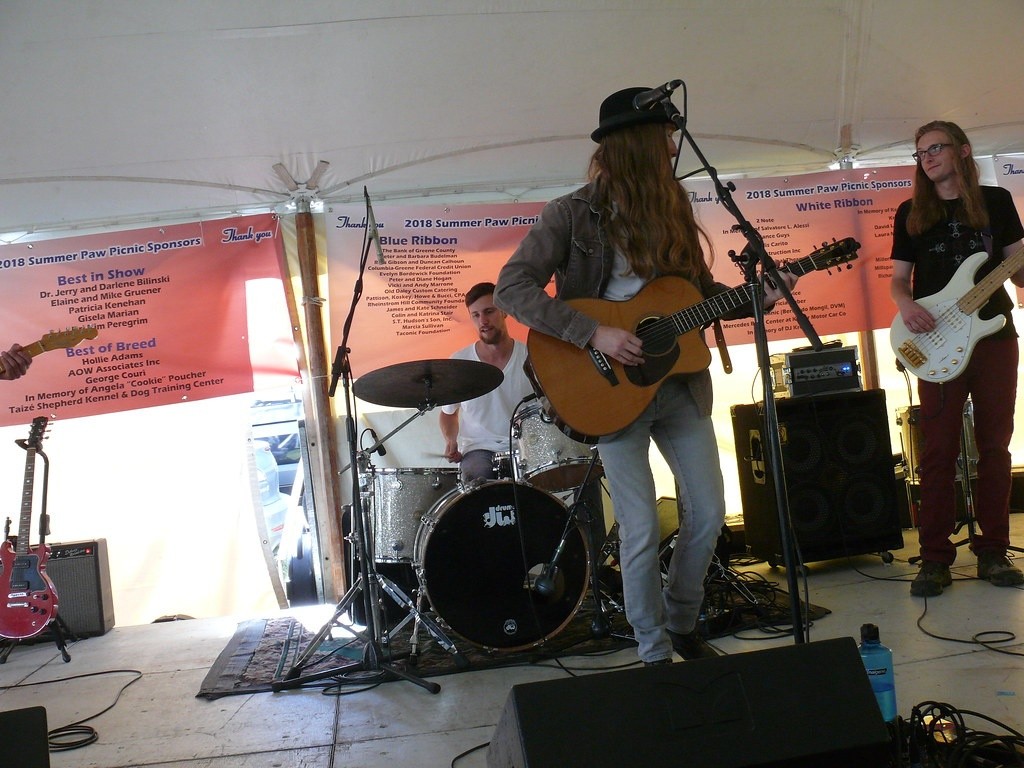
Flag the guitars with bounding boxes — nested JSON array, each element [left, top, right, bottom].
[[524, 237, 862, 445], [0, 323, 99, 373], [889, 243, 1024, 385], [0, 417, 59, 639]]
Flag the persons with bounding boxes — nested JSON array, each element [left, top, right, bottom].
[[491, 87, 797, 668], [436, 282, 545, 484], [889, 119, 1024, 597]]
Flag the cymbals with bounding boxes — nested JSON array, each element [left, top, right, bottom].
[[352, 358, 505, 409]]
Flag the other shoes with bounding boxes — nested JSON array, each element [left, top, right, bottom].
[[643, 657, 673, 667], [666, 627, 719, 660]]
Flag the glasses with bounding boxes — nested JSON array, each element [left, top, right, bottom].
[[912, 143, 954, 162]]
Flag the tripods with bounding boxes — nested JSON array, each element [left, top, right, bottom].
[[272, 231, 473, 694]]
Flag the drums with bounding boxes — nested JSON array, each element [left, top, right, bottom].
[[358, 462, 462, 566], [412, 475, 592, 654], [507, 402, 605, 494], [893, 397, 976, 487]]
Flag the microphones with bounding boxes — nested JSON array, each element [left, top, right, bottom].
[[364, 187, 385, 265], [369, 429, 387, 456], [633, 78, 681, 113], [535, 575, 556, 596]]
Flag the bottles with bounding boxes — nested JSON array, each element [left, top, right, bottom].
[[857, 623, 897, 720]]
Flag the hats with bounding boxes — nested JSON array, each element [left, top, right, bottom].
[[591, 87, 685, 144]]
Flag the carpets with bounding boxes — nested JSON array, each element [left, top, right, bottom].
[[193, 556, 834, 701]]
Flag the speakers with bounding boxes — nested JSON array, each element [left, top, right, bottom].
[[0, 705, 50, 768], [485, 635, 901, 768], [13, 538, 116, 646], [731, 388, 904, 575], [654, 496, 736, 584]]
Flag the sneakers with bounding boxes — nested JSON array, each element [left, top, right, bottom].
[[977, 554, 1024, 587], [910, 560, 953, 596]]
